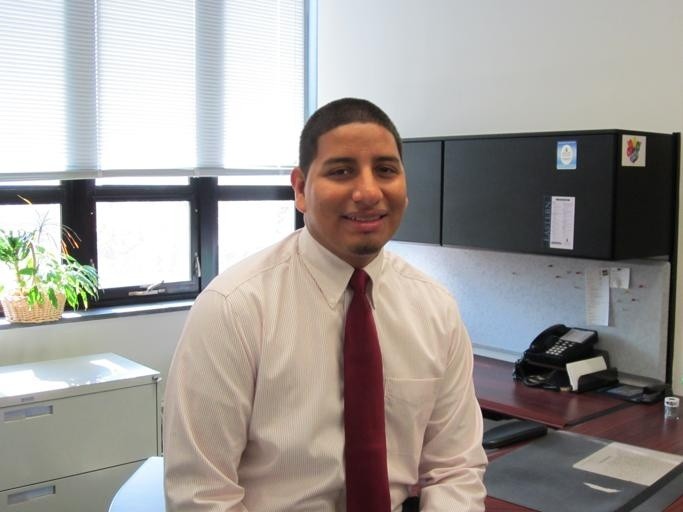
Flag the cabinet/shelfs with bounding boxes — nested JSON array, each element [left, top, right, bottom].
[[390, 127, 681, 261], [0, 352, 163, 512]]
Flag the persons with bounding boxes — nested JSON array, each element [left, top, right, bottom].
[[163, 97, 488, 512]]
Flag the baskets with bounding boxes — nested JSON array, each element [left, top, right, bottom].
[[1, 288, 67, 323]]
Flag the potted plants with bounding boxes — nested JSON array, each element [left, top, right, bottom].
[[0, 192, 107, 324]]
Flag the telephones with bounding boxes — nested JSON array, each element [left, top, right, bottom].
[[524, 324, 599, 367]]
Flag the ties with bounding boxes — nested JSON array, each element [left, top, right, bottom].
[[343, 268, 391, 512]]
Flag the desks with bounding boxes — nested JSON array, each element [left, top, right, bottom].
[[472, 347, 637, 431], [482, 396, 681, 511]]
